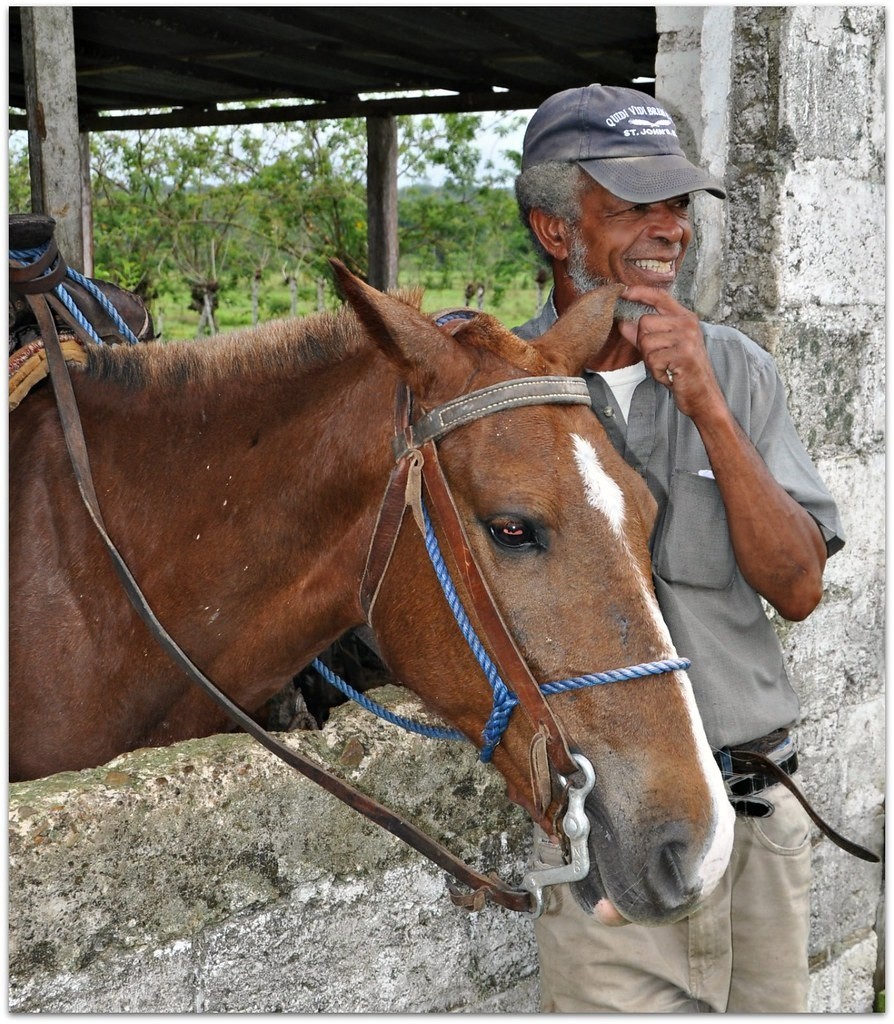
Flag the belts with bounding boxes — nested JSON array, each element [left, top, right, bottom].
[[728, 750, 799, 818]]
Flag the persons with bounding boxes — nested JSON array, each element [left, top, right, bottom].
[[503, 83, 847, 1012]]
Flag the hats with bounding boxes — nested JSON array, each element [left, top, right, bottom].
[[521, 83, 726, 203]]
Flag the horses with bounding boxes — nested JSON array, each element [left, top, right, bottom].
[[8, 212, 735, 926]]
